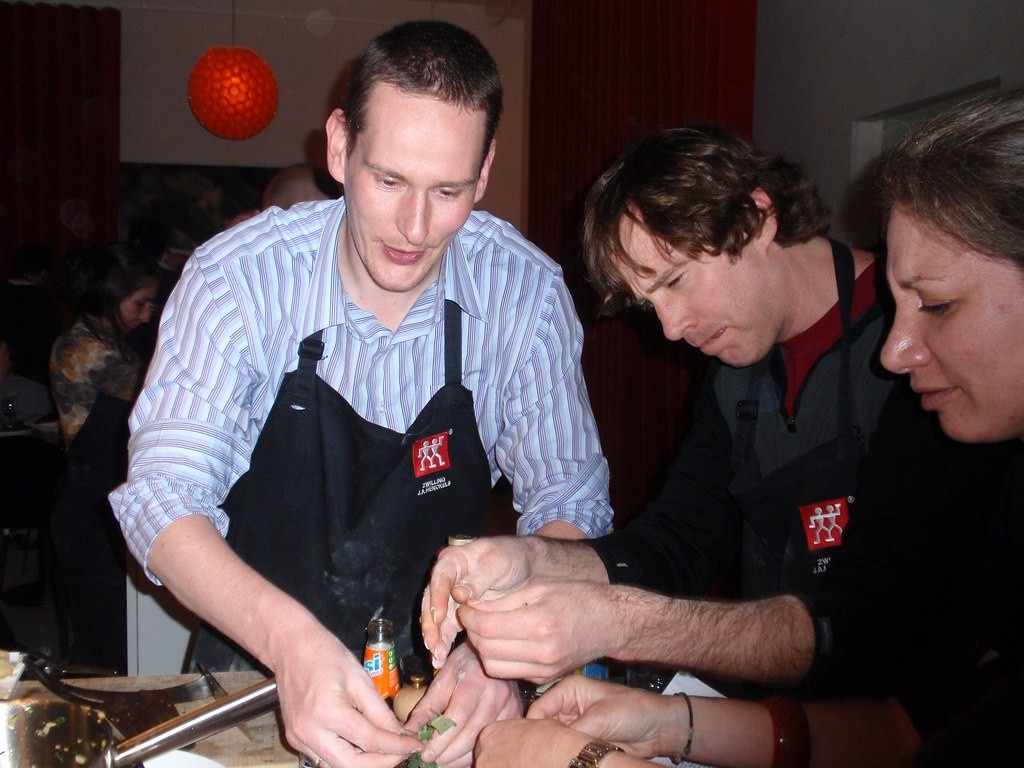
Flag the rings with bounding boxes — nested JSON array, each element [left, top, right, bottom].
[[313, 756, 323, 768]]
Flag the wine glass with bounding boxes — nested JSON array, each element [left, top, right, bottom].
[[2, 396, 18, 429]]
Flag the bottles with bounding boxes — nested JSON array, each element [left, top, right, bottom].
[[362, 619, 400, 713]]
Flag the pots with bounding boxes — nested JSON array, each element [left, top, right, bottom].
[[0, 677, 281, 768]]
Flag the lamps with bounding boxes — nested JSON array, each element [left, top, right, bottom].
[[188, 1, 280, 141]]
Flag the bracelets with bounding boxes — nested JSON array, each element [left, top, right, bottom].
[[568, 739, 625, 768], [761, 696, 811, 768], [670, 692, 694, 765]]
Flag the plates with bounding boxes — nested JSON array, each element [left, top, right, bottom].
[[24, 417, 61, 433], [0, 426, 35, 439]]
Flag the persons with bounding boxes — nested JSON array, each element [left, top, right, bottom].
[[0, 20, 1024, 768]]
